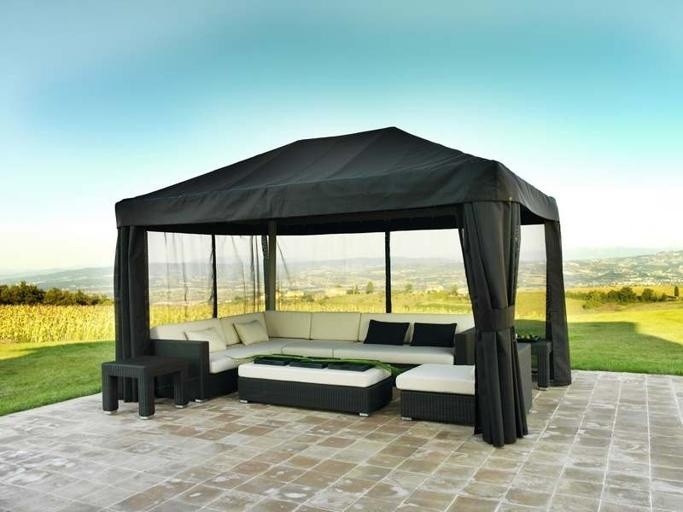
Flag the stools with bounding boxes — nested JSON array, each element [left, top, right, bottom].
[[396, 360, 476, 423], [238, 353, 393, 418]]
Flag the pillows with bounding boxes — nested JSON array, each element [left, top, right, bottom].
[[411, 322, 457, 348], [184, 326, 227, 353], [233, 319, 270, 346], [363, 319, 410, 347]]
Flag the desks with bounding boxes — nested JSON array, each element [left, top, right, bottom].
[[101, 354, 190, 420], [532, 340, 553, 390]]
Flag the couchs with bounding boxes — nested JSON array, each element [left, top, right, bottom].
[[149, 310, 532, 403]]
